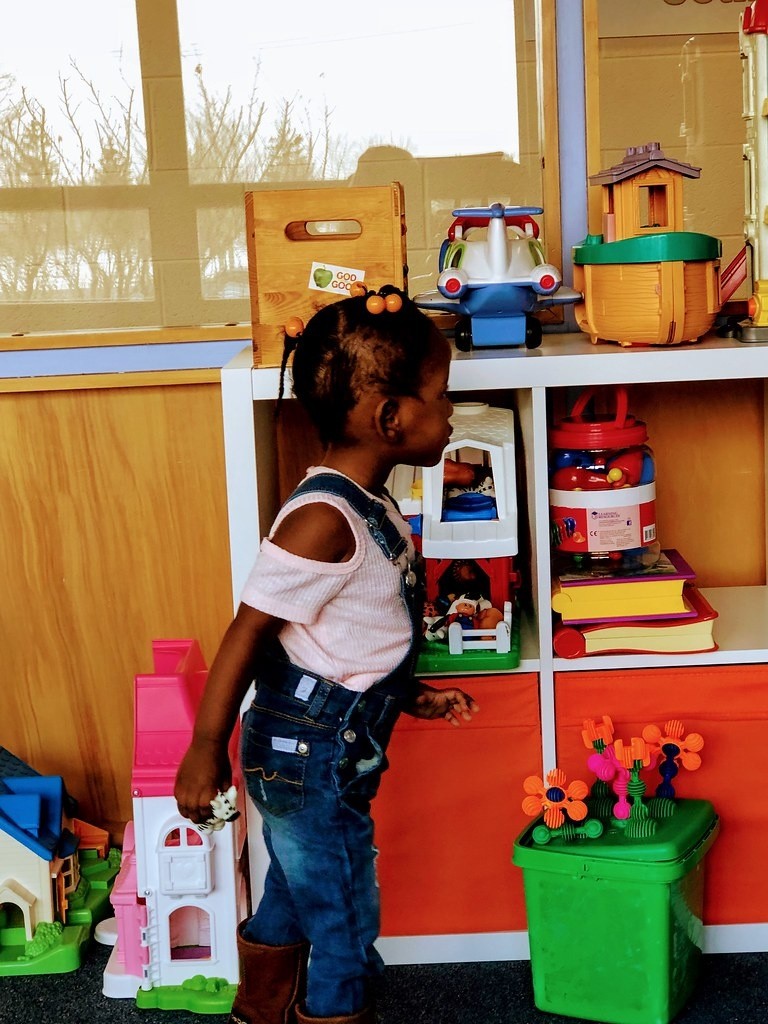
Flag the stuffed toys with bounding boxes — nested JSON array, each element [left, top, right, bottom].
[[178, 785, 241, 836]]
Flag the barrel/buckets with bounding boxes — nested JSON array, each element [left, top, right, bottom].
[[548, 384, 661, 578]]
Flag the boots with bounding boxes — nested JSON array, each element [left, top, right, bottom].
[[228, 916, 311, 1024], [295, 1000, 360, 1024]]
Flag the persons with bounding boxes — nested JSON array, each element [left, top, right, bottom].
[[176, 285, 480, 1023]]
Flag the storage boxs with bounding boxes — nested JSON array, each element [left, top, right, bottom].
[[244, 182, 407, 361]]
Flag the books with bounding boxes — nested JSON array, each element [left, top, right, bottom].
[[551, 547, 720, 660]]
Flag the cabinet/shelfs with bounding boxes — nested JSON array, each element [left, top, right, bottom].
[[222, 342, 768, 966]]
